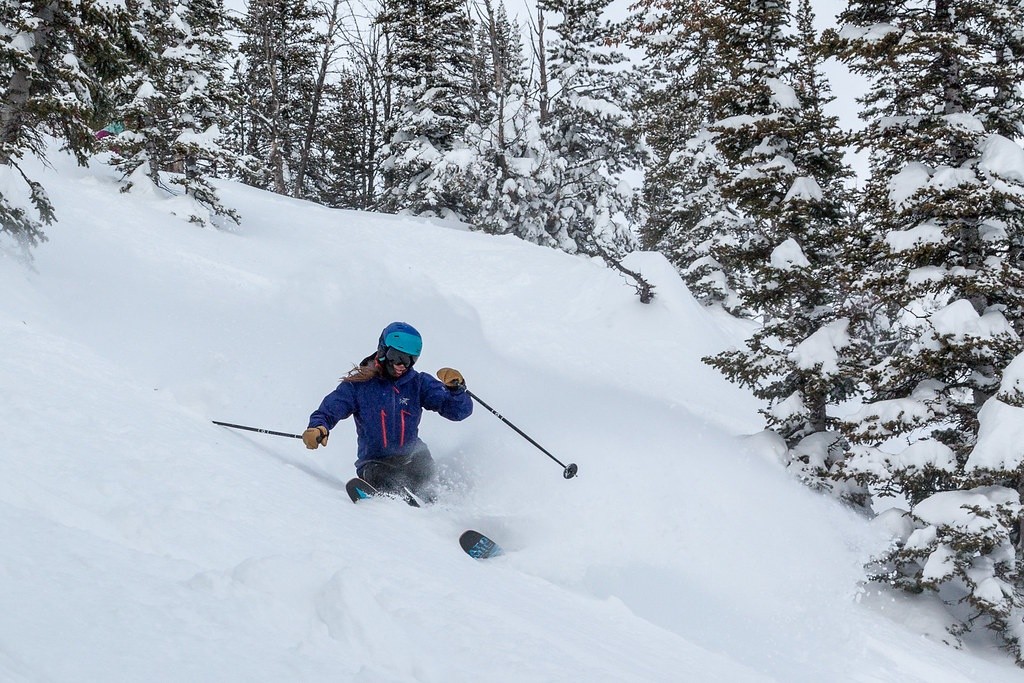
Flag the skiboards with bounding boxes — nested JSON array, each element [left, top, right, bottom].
[[342, 476, 506, 563]]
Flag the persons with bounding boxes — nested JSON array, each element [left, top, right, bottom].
[[94, 110, 185, 173], [302, 322, 505, 560]]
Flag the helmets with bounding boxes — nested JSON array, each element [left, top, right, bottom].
[[377, 322, 422, 369]]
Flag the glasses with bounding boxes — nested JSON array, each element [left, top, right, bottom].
[[385, 346, 418, 370]]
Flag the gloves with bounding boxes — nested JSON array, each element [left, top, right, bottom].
[[437, 368, 467, 395], [302, 426, 328, 450]]
